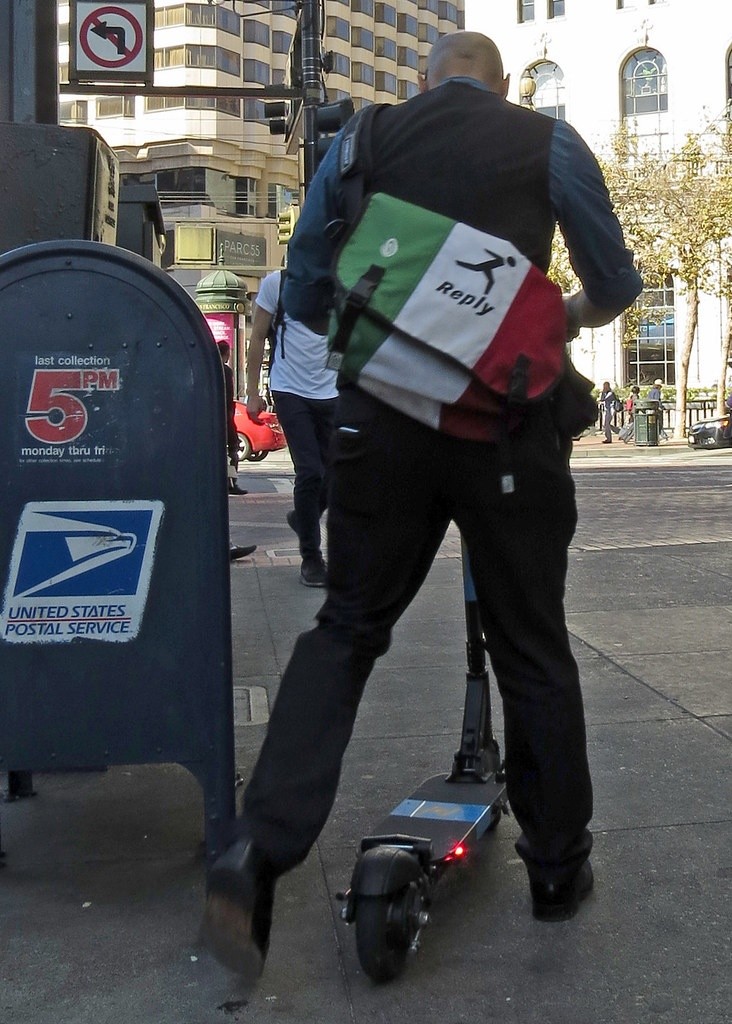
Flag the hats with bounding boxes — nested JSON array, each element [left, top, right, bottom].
[[655, 379, 664, 386]]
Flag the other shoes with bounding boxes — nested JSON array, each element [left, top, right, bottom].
[[228, 485, 248, 495], [286, 511, 297, 532], [529, 858, 594, 923], [602, 440, 612, 443], [192, 834, 274, 987], [299, 557, 329, 586], [230, 544, 257, 559]]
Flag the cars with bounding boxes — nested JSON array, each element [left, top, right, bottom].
[[234, 398, 288, 463], [687, 414, 732, 449]]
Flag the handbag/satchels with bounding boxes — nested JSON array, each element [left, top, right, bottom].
[[322, 191, 569, 442], [626, 399, 633, 411], [614, 399, 624, 412]]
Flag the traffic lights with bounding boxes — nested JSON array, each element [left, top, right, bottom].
[[316, 99, 355, 164]]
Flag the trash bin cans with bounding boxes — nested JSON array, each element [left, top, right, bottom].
[[634, 399, 660, 445], [0, 239, 243, 893]]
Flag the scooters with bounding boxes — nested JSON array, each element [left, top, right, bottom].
[[335, 327, 577, 989]]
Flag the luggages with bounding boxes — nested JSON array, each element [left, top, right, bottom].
[[618, 421, 635, 444]]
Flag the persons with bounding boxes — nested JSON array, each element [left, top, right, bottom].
[[628, 387, 647, 439], [647, 379, 666, 439], [246, 269, 340, 587], [218, 340, 248, 494], [203, 31, 644, 1002], [230, 536, 256, 560], [725, 395, 732, 410], [600, 382, 625, 443]]
